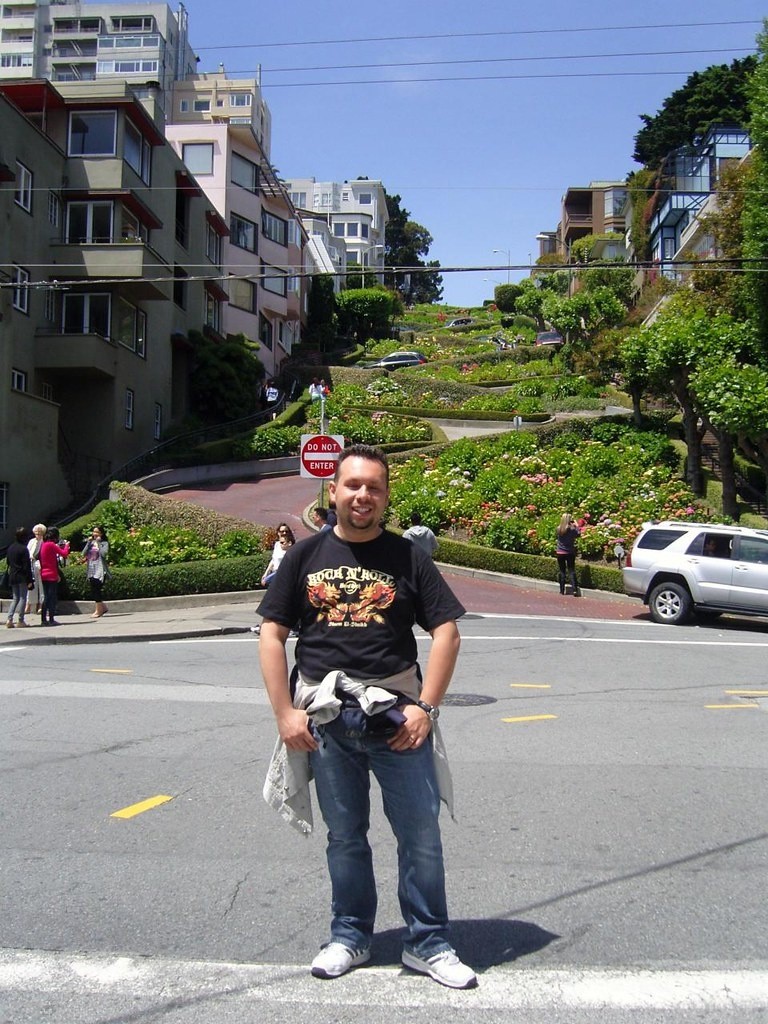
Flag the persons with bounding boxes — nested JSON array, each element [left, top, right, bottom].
[[82, 526, 112, 618], [556, 513, 582, 596], [38, 527, 71, 625], [250, 500, 339, 637], [257, 375, 328, 408], [402, 512, 439, 564], [24, 523, 47, 615], [5, 527, 33, 627], [253, 448, 478, 990]]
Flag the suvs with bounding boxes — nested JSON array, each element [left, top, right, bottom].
[[620, 519, 768, 625], [363, 351, 429, 371], [444, 317, 476, 328], [534, 330, 565, 348]]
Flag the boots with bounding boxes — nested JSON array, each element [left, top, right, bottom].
[[91, 601, 108, 618]]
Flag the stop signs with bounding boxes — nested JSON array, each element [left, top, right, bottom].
[[299, 433, 344, 478]]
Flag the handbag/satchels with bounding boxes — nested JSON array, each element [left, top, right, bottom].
[[0, 571, 10, 588]]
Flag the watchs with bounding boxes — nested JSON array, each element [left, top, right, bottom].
[[417, 700, 440, 721]]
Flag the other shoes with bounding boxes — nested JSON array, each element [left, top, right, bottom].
[[288, 631, 300, 638], [7, 622, 14, 629], [251, 626, 261, 635], [574, 592, 578, 596], [40, 620, 60, 627], [17, 621, 29, 627], [37, 609, 42, 614], [24, 610, 30, 614]]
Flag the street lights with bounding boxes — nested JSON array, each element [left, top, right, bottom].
[[492, 249, 511, 283], [536, 235, 571, 297], [483, 278, 503, 286]]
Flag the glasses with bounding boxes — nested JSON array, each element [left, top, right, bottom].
[[280, 541, 290, 545], [279, 530, 289, 533]]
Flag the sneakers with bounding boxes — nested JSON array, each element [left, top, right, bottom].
[[404, 947, 475, 990], [310, 939, 367, 980]]
